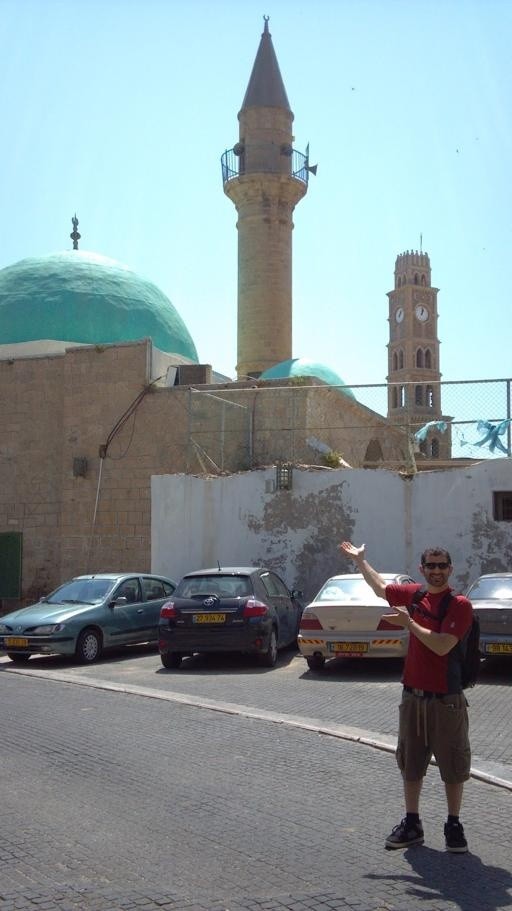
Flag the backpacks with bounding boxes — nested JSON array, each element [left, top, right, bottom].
[[413, 585, 483, 691]]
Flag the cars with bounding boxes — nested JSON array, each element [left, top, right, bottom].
[[299, 572, 416, 675], [156, 563, 306, 668], [1, 571, 179, 667], [459, 571, 512, 661]]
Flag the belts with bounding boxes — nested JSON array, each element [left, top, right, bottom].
[[402, 685, 449, 700]]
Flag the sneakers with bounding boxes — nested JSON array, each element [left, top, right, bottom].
[[384, 816, 425, 848], [444, 822, 468, 854]]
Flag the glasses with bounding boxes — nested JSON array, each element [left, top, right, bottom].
[[422, 561, 451, 570]]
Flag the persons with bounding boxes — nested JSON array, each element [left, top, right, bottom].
[[338, 539, 475, 856]]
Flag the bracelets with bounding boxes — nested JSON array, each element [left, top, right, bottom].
[[406, 620, 415, 629]]
